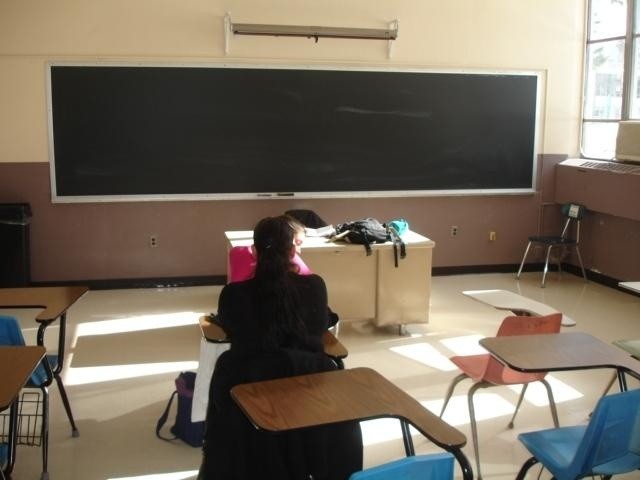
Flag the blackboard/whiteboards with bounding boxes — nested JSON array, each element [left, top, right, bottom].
[[48, 62, 540, 205]]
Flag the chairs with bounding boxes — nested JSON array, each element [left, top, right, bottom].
[[352, 452, 455, 479], [516, 387, 640, 479], [1, 315, 78, 478], [438, 313, 560, 479], [515, 203, 589, 288]]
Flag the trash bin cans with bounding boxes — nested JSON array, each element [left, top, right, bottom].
[[0, 203, 34, 288]]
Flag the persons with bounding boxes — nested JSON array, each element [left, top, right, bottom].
[[227, 212, 315, 283], [199, 215, 365, 479]]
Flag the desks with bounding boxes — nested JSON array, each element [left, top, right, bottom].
[[480, 329, 640, 383], [618, 280, 640, 294], [0, 285, 89, 322], [199, 315, 349, 368], [233, 366, 476, 479], [0, 344, 47, 410], [461, 288, 578, 327]]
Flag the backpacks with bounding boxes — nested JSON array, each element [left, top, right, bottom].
[[336, 218, 389, 243]]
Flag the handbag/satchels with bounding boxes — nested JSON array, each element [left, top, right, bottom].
[[171, 371, 204, 447]]
